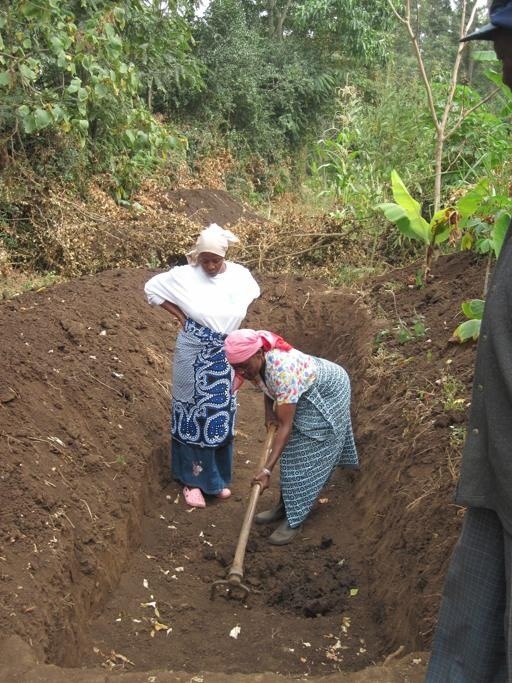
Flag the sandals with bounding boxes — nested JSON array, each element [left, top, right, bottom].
[[182, 486, 206, 510], [215, 487, 233, 498]]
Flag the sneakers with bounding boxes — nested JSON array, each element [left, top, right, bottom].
[[254, 504, 285, 523], [269, 519, 305, 545]]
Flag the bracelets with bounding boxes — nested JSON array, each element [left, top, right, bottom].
[[262, 468, 272, 476]]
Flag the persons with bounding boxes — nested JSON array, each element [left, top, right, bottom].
[[224, 328, 359, 546], [422, 0, 512, 683], [143, 223, 261, 508]]
